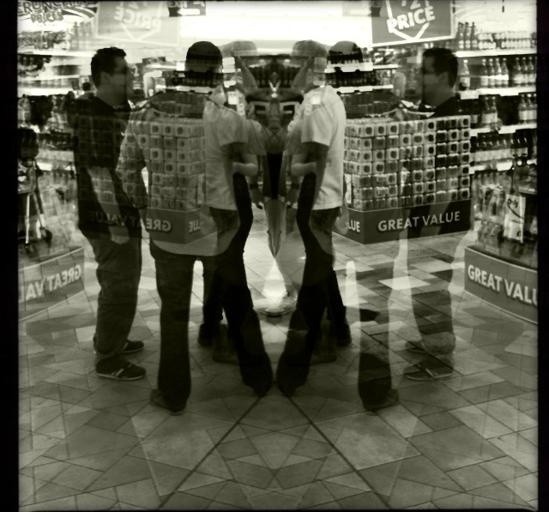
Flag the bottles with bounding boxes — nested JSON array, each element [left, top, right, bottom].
[[456, 20, 539, 267], [238, 55, 378, 90]]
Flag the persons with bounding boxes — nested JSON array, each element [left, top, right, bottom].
[[74, 39, 472, 416]]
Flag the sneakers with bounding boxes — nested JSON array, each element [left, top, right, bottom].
[[403, 361, 454, 382], [362, 387, 400, 412], [264, 293, 300, 317], [198, 321, 274, 399], [95, 356, 147, 382], [272, 297, 353, 396], [119, 339, 145, 354], [149, 387, 187, 413], [403, 336, 436, 354]]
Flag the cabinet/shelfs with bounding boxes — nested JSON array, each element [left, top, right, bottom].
[[16, 76, 97, 172], [426, 27, 536, 273]]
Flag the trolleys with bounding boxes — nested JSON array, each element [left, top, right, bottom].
[[19, 159, 54, 256]]
